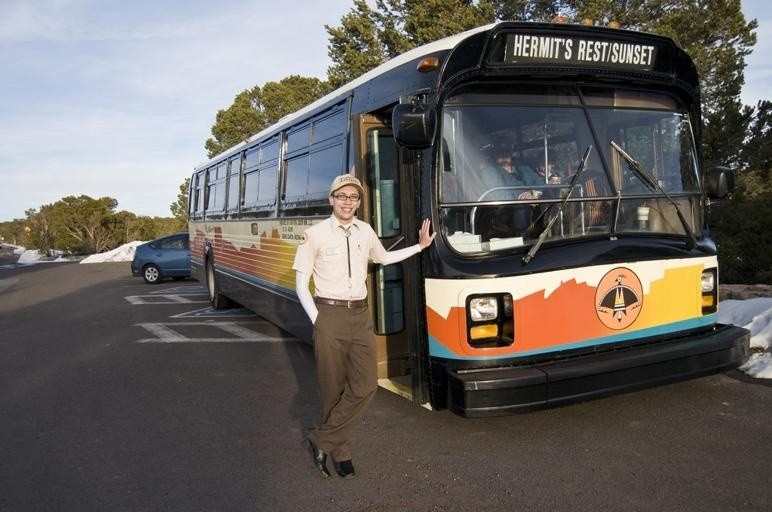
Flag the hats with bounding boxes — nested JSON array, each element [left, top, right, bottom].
[[331, 174, 365, 193]]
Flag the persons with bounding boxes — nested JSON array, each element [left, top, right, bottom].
[[292, 174, 437, 478], [466, 124, 545, 241], [523, 148, 562, 185]]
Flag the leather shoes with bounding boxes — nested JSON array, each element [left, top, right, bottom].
[[335, 460, 356, 479], [304, 428, 330, 479]]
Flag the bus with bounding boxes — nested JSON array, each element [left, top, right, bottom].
[[188, 14, 752, 418]]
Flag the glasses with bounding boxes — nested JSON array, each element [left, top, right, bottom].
[[332, 193, 361, 202]]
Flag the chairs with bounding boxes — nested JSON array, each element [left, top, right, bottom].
[[574, 170, 616, 226]]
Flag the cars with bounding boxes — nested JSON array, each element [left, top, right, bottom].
[[131, 232, 189, 284]]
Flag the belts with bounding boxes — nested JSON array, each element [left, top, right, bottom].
[[313, 296, 368, 309]]
